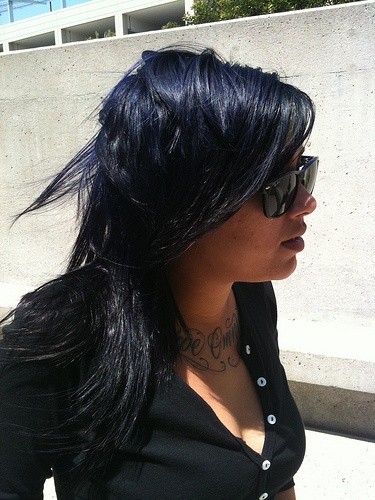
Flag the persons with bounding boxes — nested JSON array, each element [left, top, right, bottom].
[[0, 43, 319, 500]]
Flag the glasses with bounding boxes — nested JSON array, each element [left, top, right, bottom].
[[255, 154, 320, 220]]
